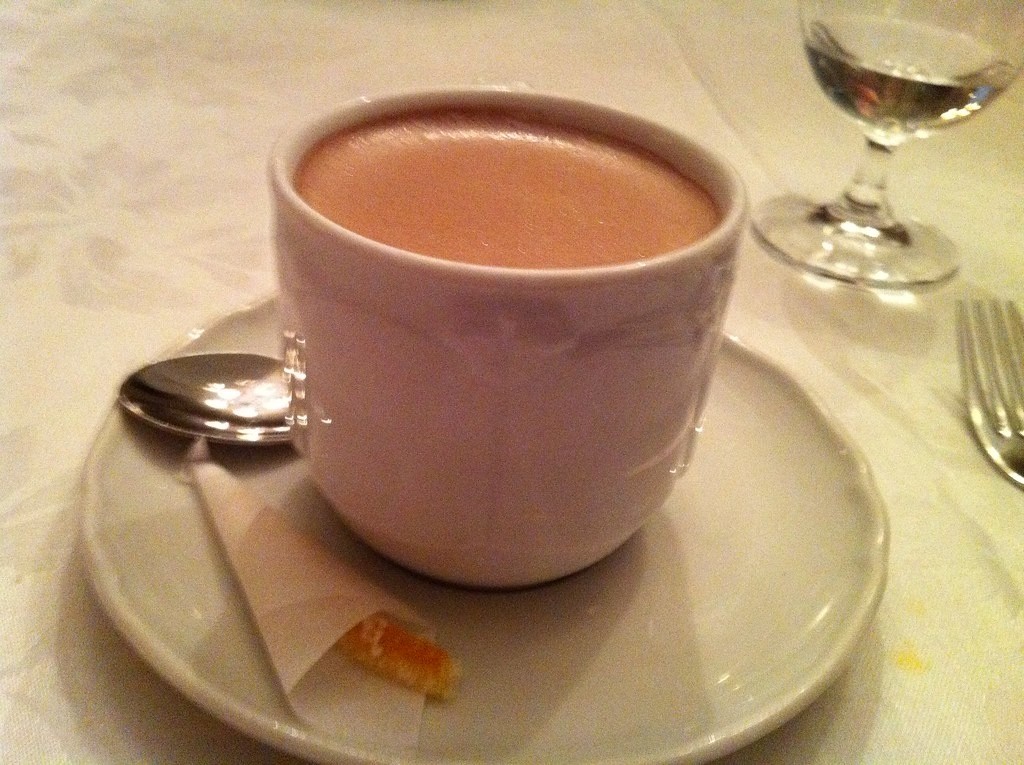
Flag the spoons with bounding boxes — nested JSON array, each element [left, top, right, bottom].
[[118, 353, 291, 445]]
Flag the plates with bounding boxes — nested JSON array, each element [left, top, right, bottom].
[[83, 287, 891, 765]]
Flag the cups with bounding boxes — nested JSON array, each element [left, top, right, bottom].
[[269, 84, 750, 590]]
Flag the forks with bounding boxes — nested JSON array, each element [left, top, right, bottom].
[[956, 297, 1024, 488]]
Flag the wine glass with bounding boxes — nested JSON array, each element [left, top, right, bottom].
[[751, 0, 1024, 291]]
